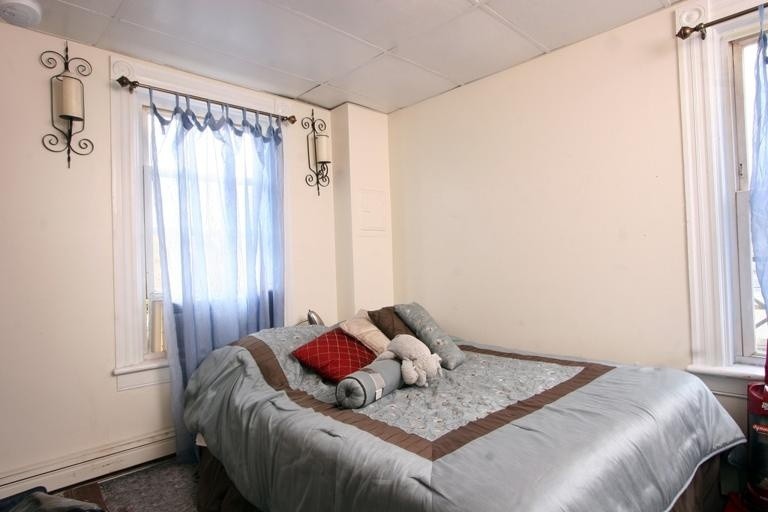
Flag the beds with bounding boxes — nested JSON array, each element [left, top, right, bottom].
[[181, 324, 748, 512]]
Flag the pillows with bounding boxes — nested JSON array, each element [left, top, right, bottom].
[[394, 301, 465, 372], [335, 359, 404, 411], [366, 306, 417, 342], [290, 327, 375, 385], [338, 309, 390, 357]]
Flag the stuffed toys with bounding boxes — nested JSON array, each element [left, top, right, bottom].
[[376, 332, 442, 391]]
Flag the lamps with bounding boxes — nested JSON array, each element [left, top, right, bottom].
[[301, 107, 333, 198], [40, 39, 94, 168]]
